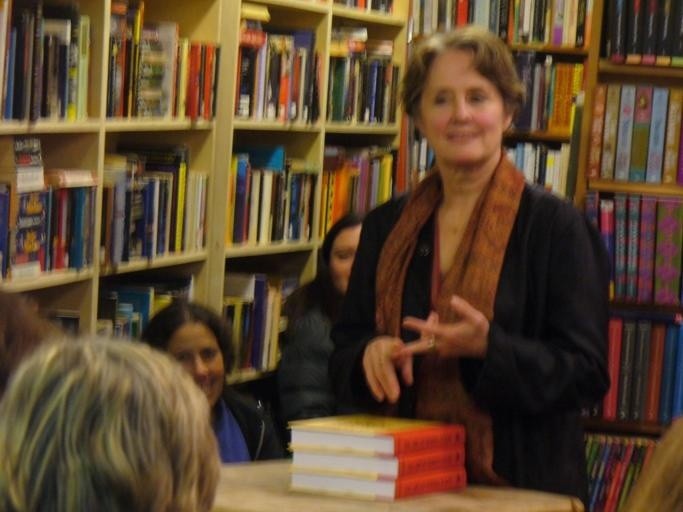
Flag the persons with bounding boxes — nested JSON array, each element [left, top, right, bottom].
[[267, 214, 364, 435], [140, 298, 285, 462], [326, 28, 613, 512], [0, 328, 222, 512]]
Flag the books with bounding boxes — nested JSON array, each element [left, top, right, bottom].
[[223, 1, 404, 374], [286, 414, 466, 501], [408, 0, 683, 512], [0, 1, 222, 346]]
